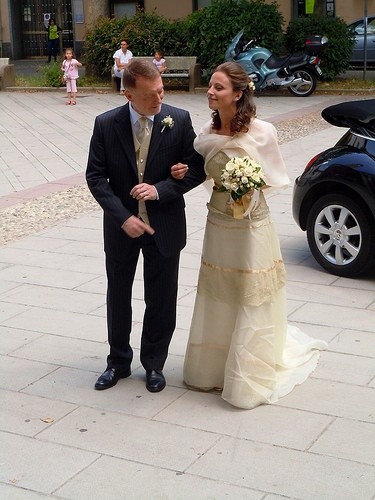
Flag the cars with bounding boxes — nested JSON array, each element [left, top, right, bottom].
[[291, 98, 375, 276], [333, 17, 375, 67]]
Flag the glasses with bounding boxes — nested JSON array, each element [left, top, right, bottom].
[[121, 44, 125, 45]]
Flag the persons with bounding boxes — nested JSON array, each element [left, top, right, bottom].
[[113, 39, 133, 95], [61, 48, 82, 106], [85, 59, 206, 392], [171, 62, 328, 409], [45, 18, 63, 63], [153, 50, 167, 74]]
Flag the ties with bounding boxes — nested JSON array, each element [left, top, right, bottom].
[[136, 116, 150, 145]]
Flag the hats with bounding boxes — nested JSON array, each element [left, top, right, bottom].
[[49, 18, 56, 25]]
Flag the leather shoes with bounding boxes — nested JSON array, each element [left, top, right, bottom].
[[145, 367, 166, 393], [95, 364, 132, 390]]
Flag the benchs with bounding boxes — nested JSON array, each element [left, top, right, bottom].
[[0, 58, 16, 90], [111, 56, 201, 94]]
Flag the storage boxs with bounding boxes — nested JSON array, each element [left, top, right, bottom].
[[305, 35, 328, 52]]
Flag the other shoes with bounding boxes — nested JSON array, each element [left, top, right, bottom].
[[47, 60, 51, 63], [120, 90, 125, 94], [72, 101, 76, 105], [68, 100, 71, 105]]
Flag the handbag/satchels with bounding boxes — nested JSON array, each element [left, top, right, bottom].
[[61, 74, 68, 84]]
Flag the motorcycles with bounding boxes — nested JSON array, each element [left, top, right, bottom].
[[225, 26, 329, 97]]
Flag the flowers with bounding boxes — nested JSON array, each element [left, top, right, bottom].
[[248, 81, 255, 90], [216, 156, 266, 204], [160, 114, 174, 133]]
